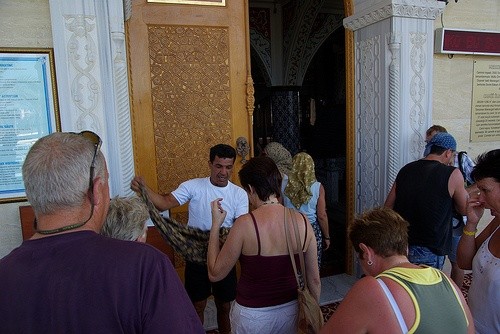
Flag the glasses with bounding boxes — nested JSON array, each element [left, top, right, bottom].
[[66, 130, 102, 195]]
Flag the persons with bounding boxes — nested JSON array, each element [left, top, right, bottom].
[[425, 125, 476, 267], [281, 152, 331, 290], [318, 206, 475, 334], [99, 196, 149, 244], [225, 156, 322, 334], [456, 149, 500, 334], [131, 144, 249, 334], [0, 131, 206, 334], [383, 132, 470, 271], [264, 142, 292, 207]]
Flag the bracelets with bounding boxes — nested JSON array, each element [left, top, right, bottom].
[[324, 237, 330, 241], [463, 227, 477, 237]]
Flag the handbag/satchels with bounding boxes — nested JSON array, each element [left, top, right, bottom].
[[297, 287, 326, 334]]
